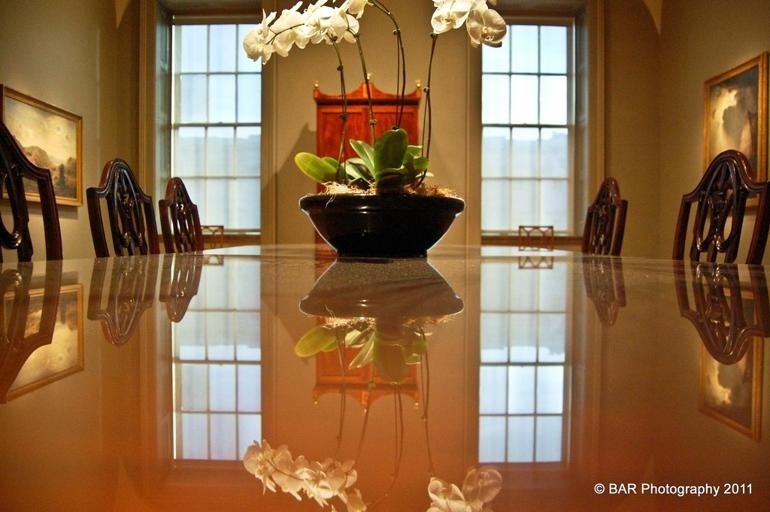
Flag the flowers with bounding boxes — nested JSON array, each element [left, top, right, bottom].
[[242, 321, 505, 511], [240, 1, 510, 188]]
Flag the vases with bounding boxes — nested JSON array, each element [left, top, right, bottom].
[[297, 260, 466, 321], [296, 190, 467, 260]]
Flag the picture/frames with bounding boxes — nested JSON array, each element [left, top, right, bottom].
[[702, 50, 770, 211], [1, 82, 85, 209], [2, 282, 86, 405], [696, 286, 766, 444]]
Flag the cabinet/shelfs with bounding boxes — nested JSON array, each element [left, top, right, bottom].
[[312, 72, 423, 243], [309, 257, 422, 414]]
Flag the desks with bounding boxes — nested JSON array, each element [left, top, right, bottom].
[[1, 240, 770, 510]]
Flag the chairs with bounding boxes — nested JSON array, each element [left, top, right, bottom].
[[157, 175, 206, 253], [1, 119, 64, 261], [580, 176, 630, 255], [583, 255, 629, 327], [87, 255, 159, 347], [670, 148, 769, 265], [671, 259, 770, 367], [85, 157, 160, 258], [157, 252, 205, 325], [1, 259, 63, 402]]
